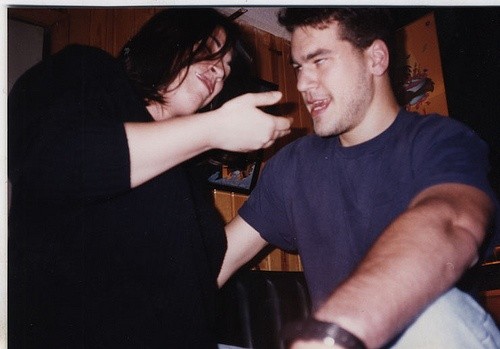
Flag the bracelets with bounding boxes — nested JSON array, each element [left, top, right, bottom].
[[285, 317, 369, 349]]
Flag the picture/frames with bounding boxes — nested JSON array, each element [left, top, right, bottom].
[[195, 77, 278, 196]]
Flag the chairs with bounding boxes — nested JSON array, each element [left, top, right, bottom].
[[216, 269, 312, 349]]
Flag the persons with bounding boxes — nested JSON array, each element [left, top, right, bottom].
[[7, 5, 294, 349], [212, 5, 500, 348]]
